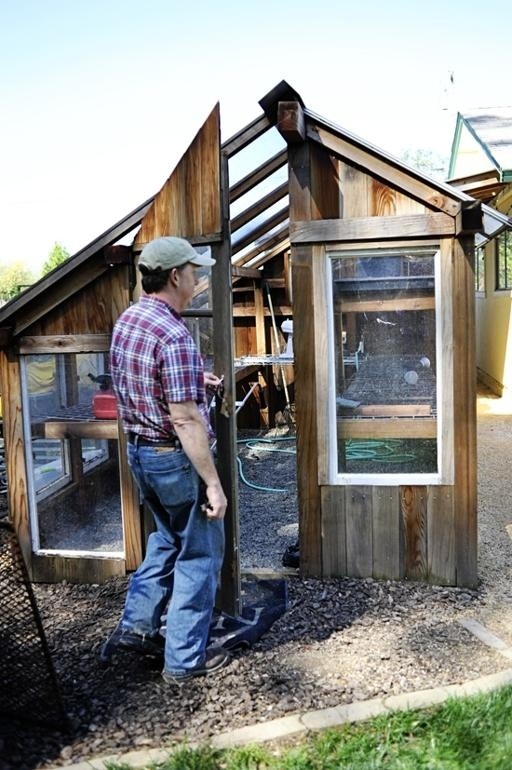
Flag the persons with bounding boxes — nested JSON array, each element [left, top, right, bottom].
[[96, 233, 236, 685]]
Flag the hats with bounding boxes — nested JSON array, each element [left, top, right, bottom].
[[137, 236, 216, 272]]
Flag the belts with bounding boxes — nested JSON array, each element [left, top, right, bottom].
[[128, 431, 181, 450]]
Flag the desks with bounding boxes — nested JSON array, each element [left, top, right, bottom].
[[336, 354, 438, 473], [30, 351, 261, 524]]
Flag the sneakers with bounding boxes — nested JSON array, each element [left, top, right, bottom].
[[118, 629, 229, 685]]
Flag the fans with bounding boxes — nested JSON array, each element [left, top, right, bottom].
[[278, 318, 295, 359]]
